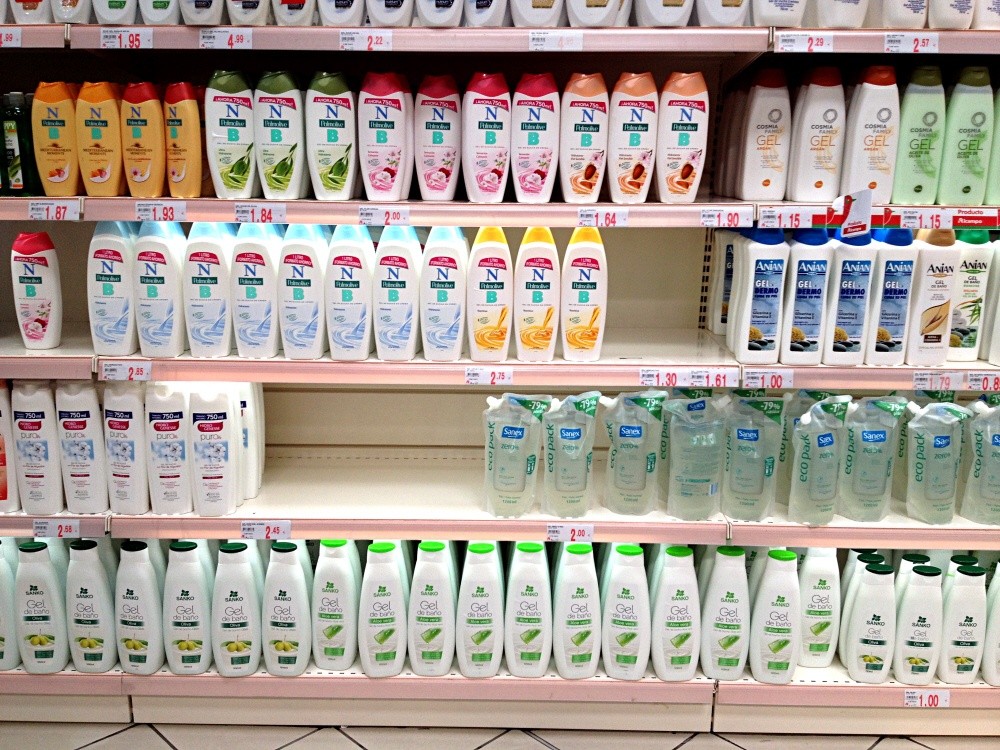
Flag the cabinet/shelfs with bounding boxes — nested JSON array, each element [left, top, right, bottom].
[[0, 16, 998, 740]]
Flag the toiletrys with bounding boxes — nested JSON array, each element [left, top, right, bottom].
[[0, 376, 268, 516], [1, 0, 1000, 371], [0, 527, 1000, 684]]
